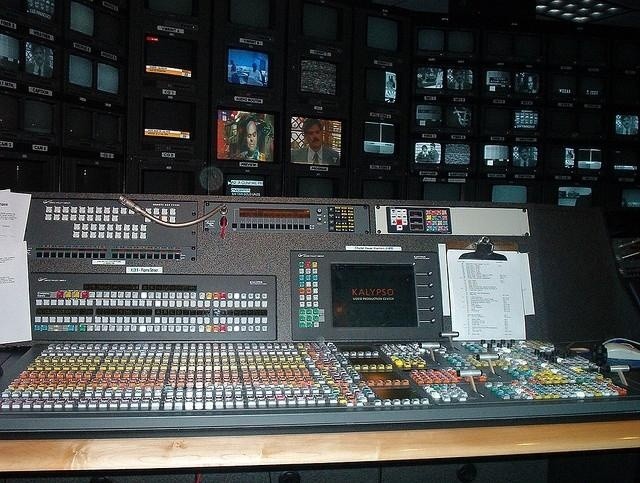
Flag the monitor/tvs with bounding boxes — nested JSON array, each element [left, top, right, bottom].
[[0, 0, 640, 209], [330, 264, 417, 327]]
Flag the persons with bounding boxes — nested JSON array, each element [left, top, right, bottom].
[[619, 115, 637, 135], [436, 69, 443, 88], [238, 119, 264, 159], [428, 143, 439, 163], [515, 147, 536, 167], [416, 145, 430, 163], [25, 42, 53, 77], [449, 69, 472, 89], [291, 117, 340, 163], [229, 57, 265, 84]]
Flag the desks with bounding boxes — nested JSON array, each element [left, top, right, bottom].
[[0, 413, 640, 483]]
